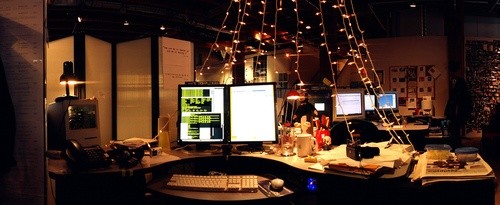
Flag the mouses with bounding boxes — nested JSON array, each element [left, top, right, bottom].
[[269, 178, 284, 191]]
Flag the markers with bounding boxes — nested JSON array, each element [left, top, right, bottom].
[[315, 115, 330, 151]]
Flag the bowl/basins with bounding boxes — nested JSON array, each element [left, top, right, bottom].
[[425, 144, 452, 159], [455, 147, 478, 160]]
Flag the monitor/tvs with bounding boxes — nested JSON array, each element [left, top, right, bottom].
[[177, 83, 227, 153], [227, 82, 278, 152], [376, 93, 399, 112], [364, 92, 376, 113], [332, 89, 365, 122], [314, 102, 325, 111]]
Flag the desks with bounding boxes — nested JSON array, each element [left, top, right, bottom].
[[377, 121, 430, 145], [47, 145, 495, 205]]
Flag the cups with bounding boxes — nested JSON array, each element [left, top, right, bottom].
[[281, 127, 295, 156], [296, 134, 317, 157]]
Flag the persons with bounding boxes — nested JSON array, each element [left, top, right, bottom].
[[292, 92, 318, 122], [444, 78, 474, 127]]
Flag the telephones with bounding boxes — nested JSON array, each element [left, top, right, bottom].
[[66, 139, 112, 168]]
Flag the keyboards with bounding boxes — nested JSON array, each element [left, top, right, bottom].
[[167, 173, 258, 192]]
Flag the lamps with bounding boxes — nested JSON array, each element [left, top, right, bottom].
[[55, 60, 82, 102], [284, 85, 300, 123]]
